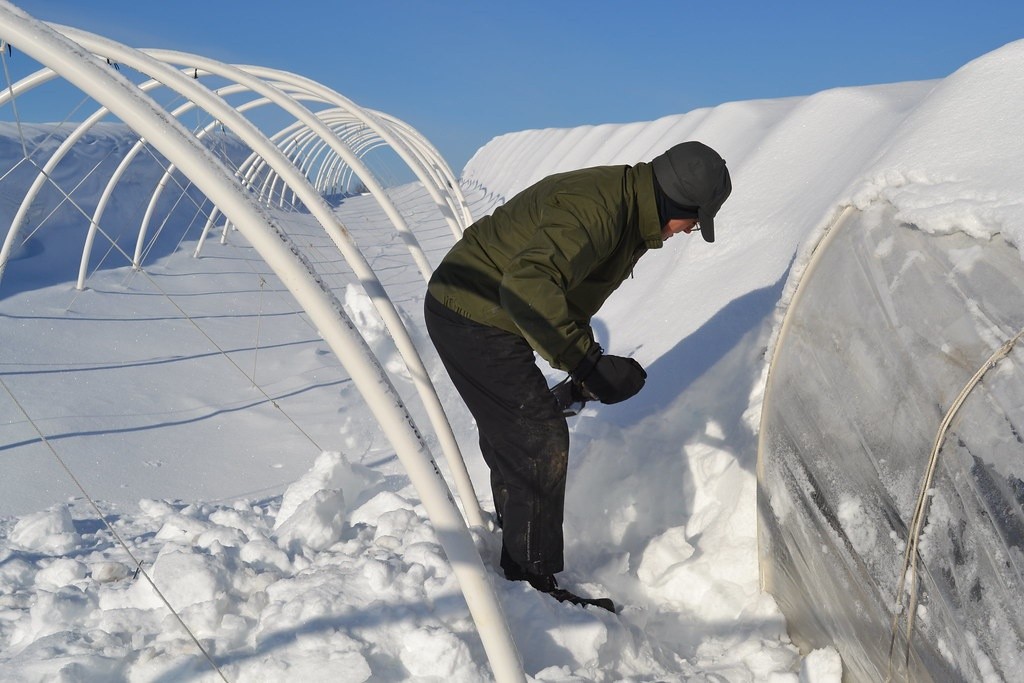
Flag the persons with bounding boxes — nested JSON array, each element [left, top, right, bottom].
[[424, 141, 732, 611]]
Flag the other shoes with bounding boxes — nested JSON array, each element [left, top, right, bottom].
[[506, 569, 614, 616]]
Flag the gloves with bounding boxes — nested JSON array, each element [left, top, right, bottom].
[[568, 345, 647, 404]]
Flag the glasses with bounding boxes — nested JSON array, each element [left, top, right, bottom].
[[692, 222, 700, 233]]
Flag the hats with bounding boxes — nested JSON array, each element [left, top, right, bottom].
[[654, 141, 733, 243]]
